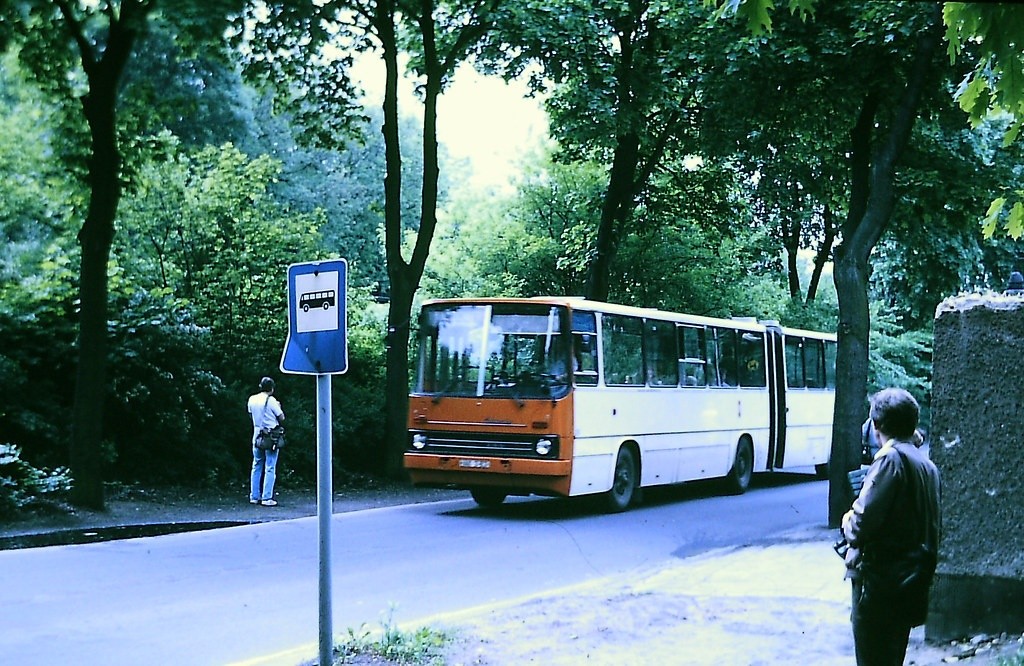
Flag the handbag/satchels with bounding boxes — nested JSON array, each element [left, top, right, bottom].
[[851, 557, 931, 631], [253, 432, 286, 449]]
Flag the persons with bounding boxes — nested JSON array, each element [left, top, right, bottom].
[[539, 348, 579, 398], [247, 376, 285, 506], [839, 388, 943, 666]]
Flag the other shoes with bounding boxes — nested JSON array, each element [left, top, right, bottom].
[[249, 498, 278, 506]]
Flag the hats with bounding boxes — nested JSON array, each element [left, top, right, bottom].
[[259, 377, 274, 384]]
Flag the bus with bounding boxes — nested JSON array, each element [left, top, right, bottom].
[[401, 294, 837, 515]]
